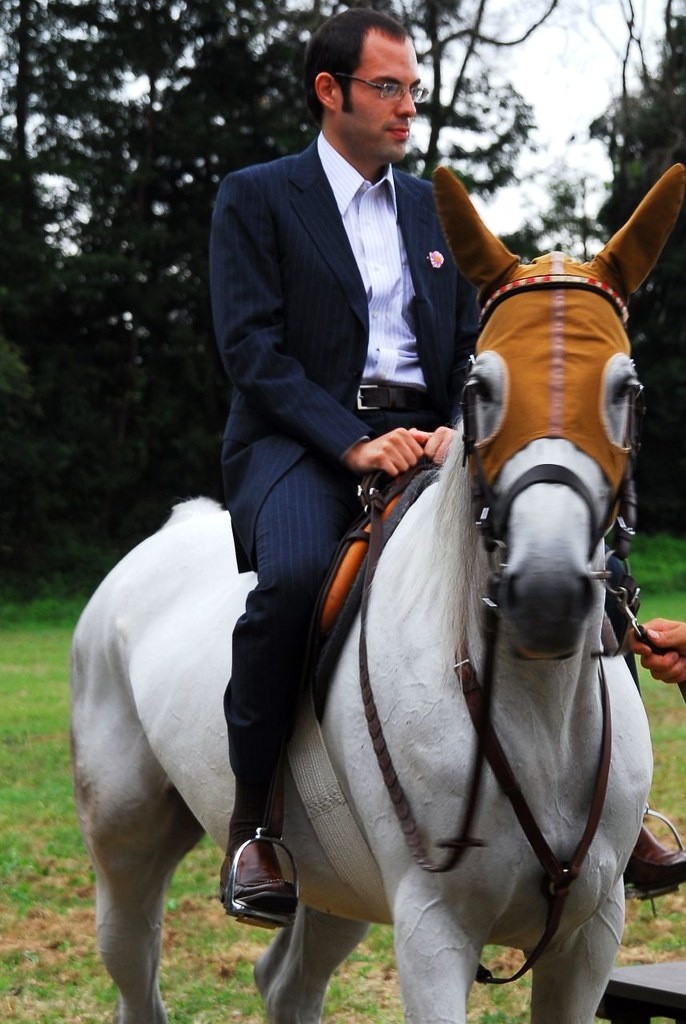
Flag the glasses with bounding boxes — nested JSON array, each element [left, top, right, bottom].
[[331, 72, 430, 104]]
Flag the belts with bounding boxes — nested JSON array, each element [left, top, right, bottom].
[[356, 385, 432, 409]]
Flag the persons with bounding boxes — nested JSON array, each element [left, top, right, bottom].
[[208, 9, 686, 912]]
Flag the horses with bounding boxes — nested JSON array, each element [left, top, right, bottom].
[[69, 160, 685, 1024]]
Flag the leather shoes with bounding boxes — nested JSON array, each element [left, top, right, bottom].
[[219, 842, 297, 914], [624, 823, 686, 887]]
